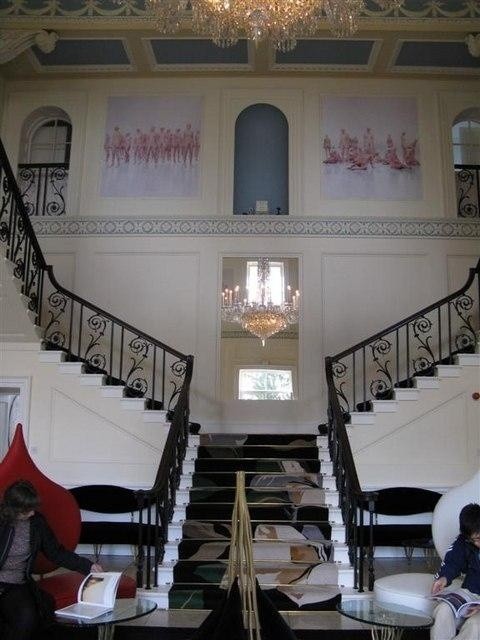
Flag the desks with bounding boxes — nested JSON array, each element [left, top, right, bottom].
[[60, 600, 157, 639], [338, 599, 435, 639]]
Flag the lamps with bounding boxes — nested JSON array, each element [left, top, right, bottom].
[[146, 1, 364, 53], [219, 256, 299, 349]]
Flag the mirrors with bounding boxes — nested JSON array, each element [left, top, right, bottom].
[[218, 253, 302, 403]]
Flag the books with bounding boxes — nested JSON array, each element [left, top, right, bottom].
[[53, 571, 123, 620], [431, 588, 480, 619]]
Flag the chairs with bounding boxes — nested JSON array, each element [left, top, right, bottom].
[[69, 485, 161, 562], [355, 487, 443, 567], [0, 422, 135, 625], [374, 470, 480, 638]]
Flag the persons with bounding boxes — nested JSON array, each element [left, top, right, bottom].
[[0, 481, 105, 640], [104, 122, 203, 168], [322, 127, 421, 170], [430, 504, 479, 639]]
